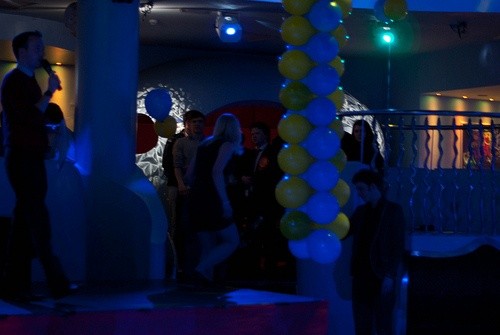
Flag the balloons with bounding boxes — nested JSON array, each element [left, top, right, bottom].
[[279, 0, 349, 95], [374, 0, 407, 24], [145, 88, 172, 121], [155, 116, 176, 138], [136, 113, 148, 154], [276, 174, 350, 264], [278, 82, 347, 175]]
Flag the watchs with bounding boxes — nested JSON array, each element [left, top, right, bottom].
[[45, 90, 52, 97]]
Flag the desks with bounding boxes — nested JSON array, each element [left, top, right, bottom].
[[406, 231, 499, 256]]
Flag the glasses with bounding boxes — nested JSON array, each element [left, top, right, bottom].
[[250, 131, 265, 136]]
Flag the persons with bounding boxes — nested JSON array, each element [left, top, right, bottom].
[[162, 110, 298, 293], [44, 102, 67, 169], [0, 30, 61, 302], [342, 119, 384, 177], [349, 168, 407, 335]]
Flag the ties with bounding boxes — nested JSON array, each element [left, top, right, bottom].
[[254, 149, 261, 171]]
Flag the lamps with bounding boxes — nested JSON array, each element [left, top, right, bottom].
[[216, 15, 242, 43]]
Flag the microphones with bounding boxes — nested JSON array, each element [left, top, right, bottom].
[[41, 59, 63, 91]]
